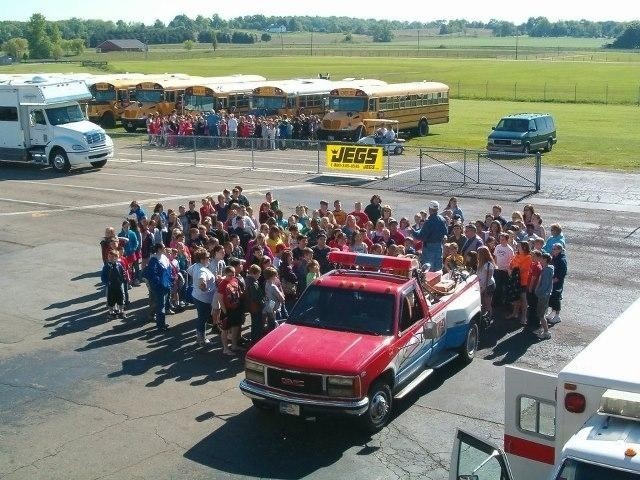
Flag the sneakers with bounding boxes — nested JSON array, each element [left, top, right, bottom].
[[545, 311, 556, 320], [536, 331, 551, 339], [532, 328, 544, 335], [549, 316, 562, 323]]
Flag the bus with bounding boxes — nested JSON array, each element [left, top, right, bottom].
[[120, 74, 267, 134], [0, 71, 188, 130], [251, 77, 387, 121], [181, 77, 331, 119], [318, 81, 449, 140]]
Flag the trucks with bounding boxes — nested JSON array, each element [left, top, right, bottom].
[[0, 73, 115, 173], [446, 298, 640, 480], [487, 112, 557, 156]]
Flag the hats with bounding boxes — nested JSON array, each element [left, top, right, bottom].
[[429, 200, 439, 210]]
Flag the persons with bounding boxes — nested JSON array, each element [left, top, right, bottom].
[[381, 125, 396, 143], [147, 109, 318, 153], [378, 123, 386, 135], [99, 184, 567, 354]]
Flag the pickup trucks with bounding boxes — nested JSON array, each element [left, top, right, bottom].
[[241, 248, 484, 430]]
